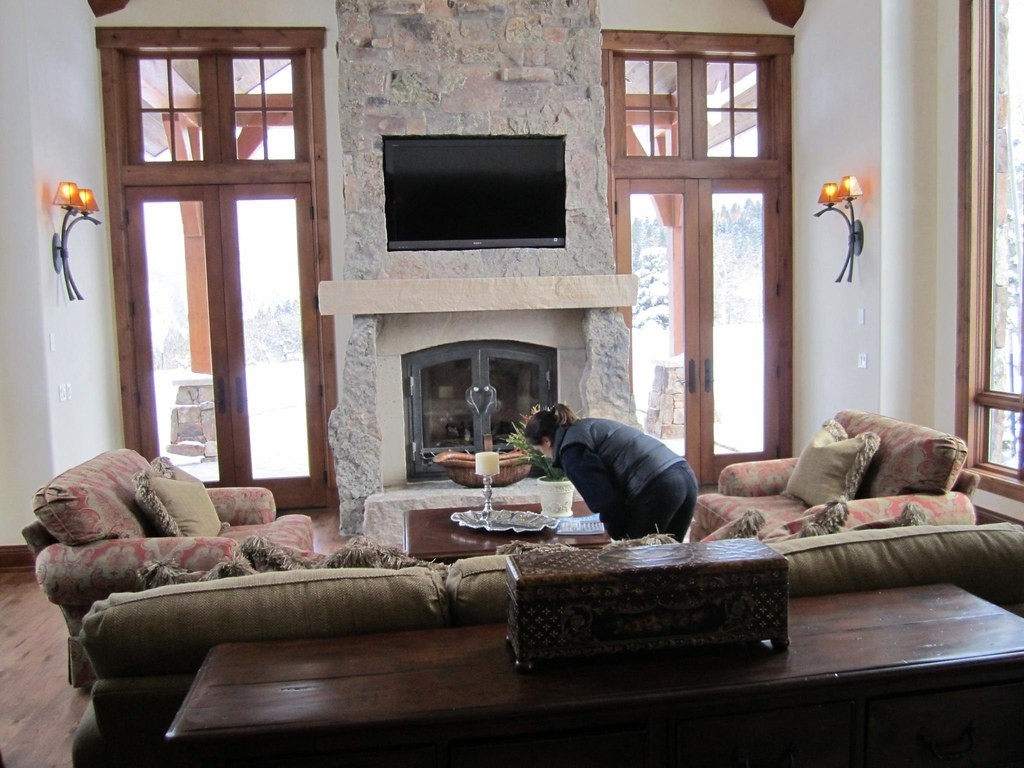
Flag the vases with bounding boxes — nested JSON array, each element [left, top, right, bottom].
[[537, 476, 575, 518]]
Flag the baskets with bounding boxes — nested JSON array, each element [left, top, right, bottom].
[[432, 450, 534, 487]]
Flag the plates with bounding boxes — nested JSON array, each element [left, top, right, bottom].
[[452, 507, 559, 534]]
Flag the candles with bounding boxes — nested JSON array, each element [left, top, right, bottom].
[[475, 449, 502, 479]]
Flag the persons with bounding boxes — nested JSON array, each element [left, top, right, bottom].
[[524, 403, 698, 543]]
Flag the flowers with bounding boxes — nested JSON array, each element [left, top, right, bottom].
[[503, 400, 585, 482]]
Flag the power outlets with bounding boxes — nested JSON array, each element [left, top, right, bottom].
[[857, 353, 867, 368]]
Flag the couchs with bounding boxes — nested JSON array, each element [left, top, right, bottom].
[[690, 406, 978, 545], [20, 447, 314, 687]]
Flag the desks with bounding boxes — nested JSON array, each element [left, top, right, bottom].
[[403, 500, 612, 563], [162, 579, 1024, 768]]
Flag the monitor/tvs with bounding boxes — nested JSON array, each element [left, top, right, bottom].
[[381, 134, 568, 252]]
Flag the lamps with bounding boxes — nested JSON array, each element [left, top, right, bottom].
[[813, 174, 864, 285], [52, 181, 104, 303]]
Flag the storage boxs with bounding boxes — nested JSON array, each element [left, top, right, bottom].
[[507, 535, 792, 671]]
